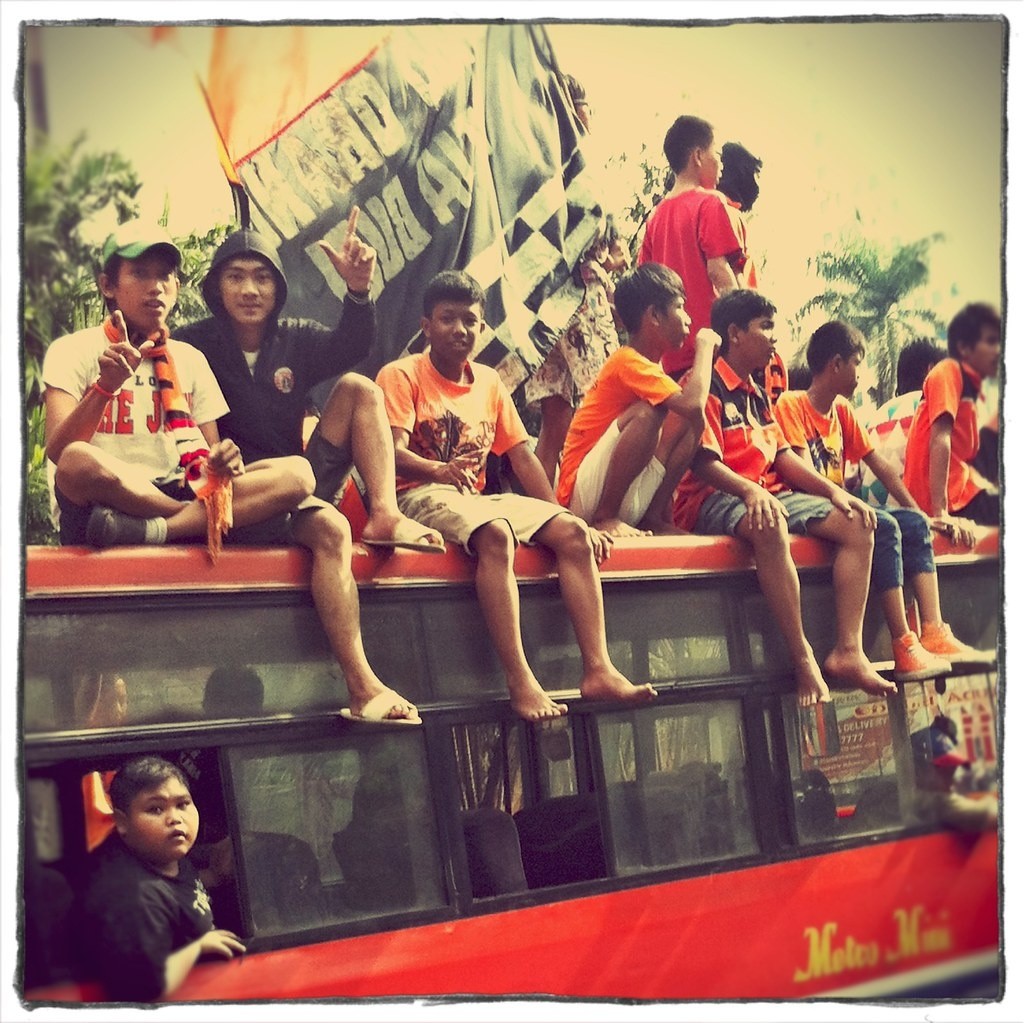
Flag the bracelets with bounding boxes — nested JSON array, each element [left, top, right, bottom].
[[91, 382, 115, 402]]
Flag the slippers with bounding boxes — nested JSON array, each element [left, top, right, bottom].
[[340, 690, 422, 724], [361, 519, 446, 554]]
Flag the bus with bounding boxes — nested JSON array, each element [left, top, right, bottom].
[[15, 522, 1003, 1005]]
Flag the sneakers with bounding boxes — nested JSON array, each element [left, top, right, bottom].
[[891, 631, 952, 681], [919, 621, 992, 672]]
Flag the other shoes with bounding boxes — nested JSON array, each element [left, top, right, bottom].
[[85, 503, 147, 547]]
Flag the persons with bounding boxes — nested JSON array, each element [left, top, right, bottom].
[[41, 75, 1006, 724], [44, 667, 263, 1002], [912, 715, 960, 778]]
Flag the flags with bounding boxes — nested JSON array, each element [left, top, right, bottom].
[[171, 25, 607, 395]]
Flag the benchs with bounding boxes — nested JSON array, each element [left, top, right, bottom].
[[331, 809, 527, 913], [241, 829, 329, 931], [511, 786, 696, 883]]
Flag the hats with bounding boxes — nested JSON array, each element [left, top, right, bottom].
[[102, 219, 183, 273]]
